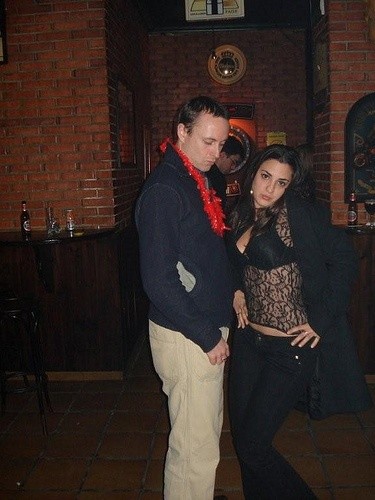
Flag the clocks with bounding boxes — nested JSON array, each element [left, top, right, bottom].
[[207, 42, 246, 85]]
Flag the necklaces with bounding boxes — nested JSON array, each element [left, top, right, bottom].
[[160, 138, 226, 238]]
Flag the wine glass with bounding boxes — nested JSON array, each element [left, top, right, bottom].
[[364, 199, 375, 230]]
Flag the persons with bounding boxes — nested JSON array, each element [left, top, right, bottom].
[[133, 97, 234, 500], [203, 135, 244, 212], [241, 144, 375, 500]]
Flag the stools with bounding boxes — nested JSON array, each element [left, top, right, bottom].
[[0, 293, 58, 439]]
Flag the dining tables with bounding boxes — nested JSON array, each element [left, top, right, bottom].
[[1, 228, 119, 250]]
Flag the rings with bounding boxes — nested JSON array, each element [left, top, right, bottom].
[[236, 313, 239, 317]]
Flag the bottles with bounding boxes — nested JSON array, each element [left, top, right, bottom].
[[347, 191, 358, 226], [20, 200, 32, 235]]
